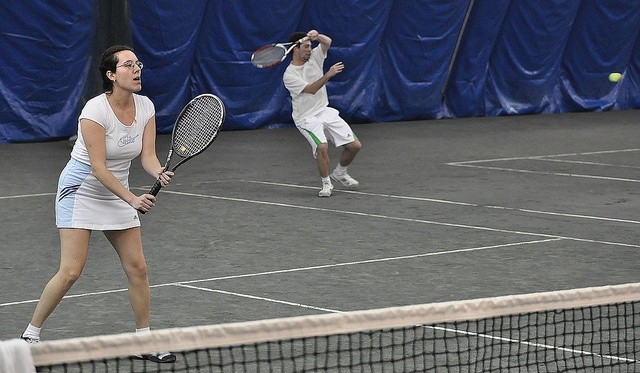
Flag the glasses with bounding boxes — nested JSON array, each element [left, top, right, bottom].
[[117, 61, 144, 69]]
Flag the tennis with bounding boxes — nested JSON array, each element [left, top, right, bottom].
[[608, 72, 623, 82]]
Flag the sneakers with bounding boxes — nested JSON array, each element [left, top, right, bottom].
[[130, 352, 176, 362], [18, 333, 40, 343], [318, 181, 334, 197], [330, 169, 360, 189]]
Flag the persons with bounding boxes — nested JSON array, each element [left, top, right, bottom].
[[21, 45, 176, 372], [283, 29, 362, 197]]
